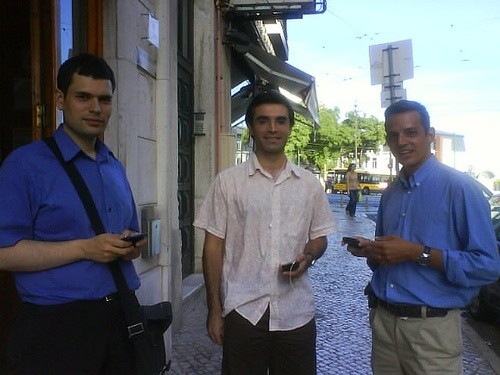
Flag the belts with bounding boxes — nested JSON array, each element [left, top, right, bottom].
[[64, 294, 114, 308], [379, 299, 448, 318]]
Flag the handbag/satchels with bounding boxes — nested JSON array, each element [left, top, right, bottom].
[[130, 298, 174, 374]]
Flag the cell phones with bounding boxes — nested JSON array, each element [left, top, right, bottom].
[[282, 262, 296, 273], [126, 232, 151, 244], [342, 236, 358, 249]]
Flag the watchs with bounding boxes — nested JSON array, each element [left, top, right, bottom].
[[416, 246, 431, 266], [303, 252, 316, 267]]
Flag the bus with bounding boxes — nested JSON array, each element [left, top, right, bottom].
[[333, 168, 396, 195]]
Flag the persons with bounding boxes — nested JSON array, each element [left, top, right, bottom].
[[0, 54, 148, 375], [345, 163, 360, 217], [193, 89, 336, 374], [341, 100, 500, 375]]
